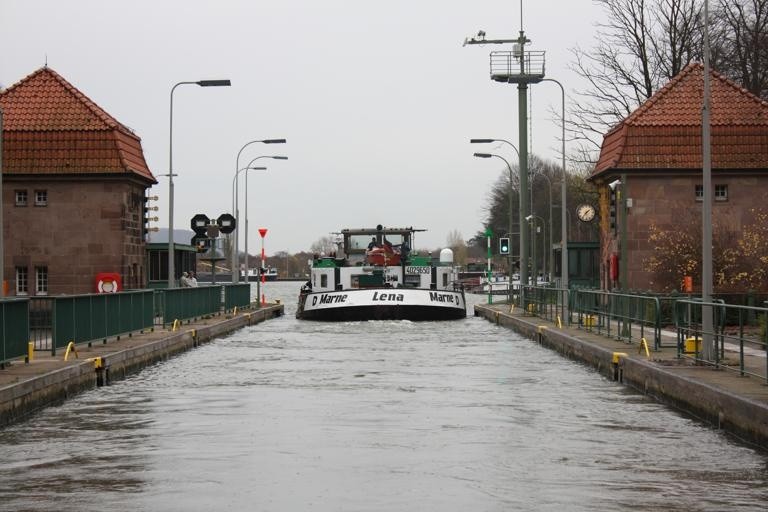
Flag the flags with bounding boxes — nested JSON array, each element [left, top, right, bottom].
[[383, 236, 394, 258]]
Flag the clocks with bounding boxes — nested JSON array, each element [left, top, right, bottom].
[[576, 202, 596, 223]]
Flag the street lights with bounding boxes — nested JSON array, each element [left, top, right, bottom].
[[147, 173, 178, 243], [231, 139, 288, 285], [474, 153, 514, 304], [508, 76, 571, 324], [526, 170, 554, 282], [471, 139, 538, 288], [168, 79, 232, 289]]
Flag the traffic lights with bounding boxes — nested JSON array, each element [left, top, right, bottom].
[[500, 238, 510, 256]]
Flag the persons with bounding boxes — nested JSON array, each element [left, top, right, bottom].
[[368, 237, 377, 250], [187, 270, 197, 287], [180, 271, 192, 287]]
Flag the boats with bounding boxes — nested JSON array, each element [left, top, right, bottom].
[[455, 260, 549, 294], [296, 225, 467, 320]]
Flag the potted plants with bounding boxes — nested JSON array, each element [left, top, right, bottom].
[[643, 210, 733, 293]]
